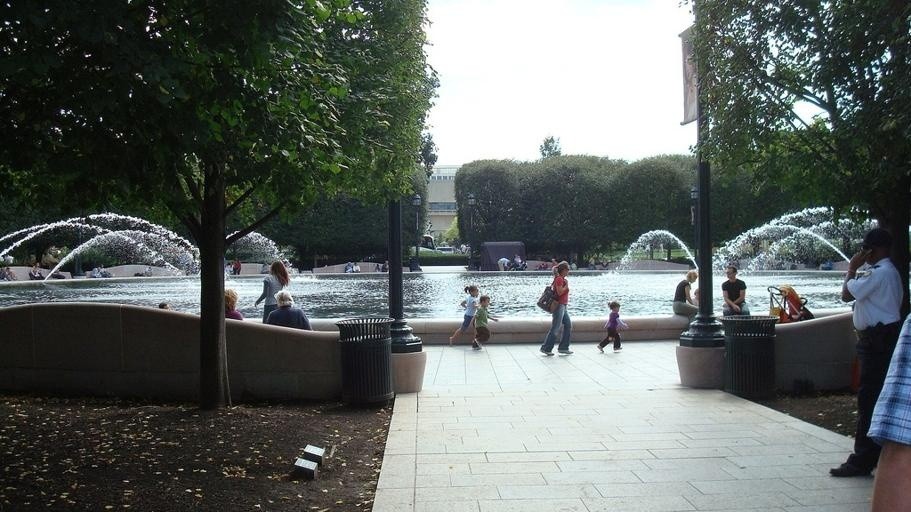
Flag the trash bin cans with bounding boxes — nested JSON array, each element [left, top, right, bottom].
[[719, 315, 779, 400], [334, 317, 396, 408]]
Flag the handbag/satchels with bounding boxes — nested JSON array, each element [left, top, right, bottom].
[[537, 277, 565, 313]]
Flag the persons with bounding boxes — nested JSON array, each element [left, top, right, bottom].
[[233, 258, 241, 275], [540, 261, 574, 356], [470, 295, 498, 349], [690, 289, 700, 307], [345, 262, 360, 273], [498, 256, 607, 271], [375, 261, 389, 272], [597, 301, 628, 353], [158, 302, 170, 310], [673, 270, 699, 324], [450, 286, 479, 346], [224, 287, 243, 320], [722, 266, 750, 315], [29, 263, 65, 279], [145, 266, 152, 277], [867, 312, 911, 512], [254, 261, 289, 322], [267, 289, 312, 331], [830, 227, 905, 477], [92, 265, 110, 278], [0, 267, 17, 280]]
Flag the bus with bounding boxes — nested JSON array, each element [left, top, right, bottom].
[[437, 247, 456, 254], [421, 235, 436, 249]]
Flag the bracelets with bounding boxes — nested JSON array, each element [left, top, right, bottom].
[[848, 270, 855, 275]]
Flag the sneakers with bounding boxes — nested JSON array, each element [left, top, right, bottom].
[[540, 348, 573, 355], [831, 454, 868, 477]]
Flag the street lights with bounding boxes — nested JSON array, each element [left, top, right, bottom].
[[412, 194, 422, 271], [468, 192, 475, 242], [689, 185, 700, 266]]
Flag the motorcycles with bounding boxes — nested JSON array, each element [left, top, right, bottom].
[[509, 255, 528, 272]]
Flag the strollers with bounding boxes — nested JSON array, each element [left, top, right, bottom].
[[766, 287, 816, 325]]
